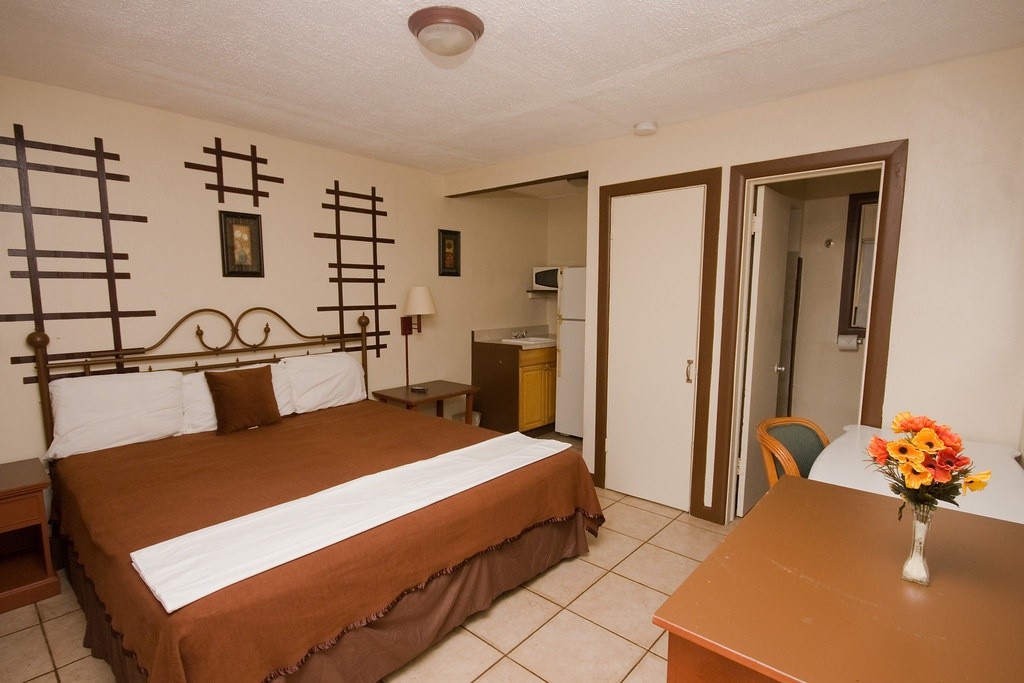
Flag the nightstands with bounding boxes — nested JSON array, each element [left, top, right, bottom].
[[370, 379, 477, 427], [0, 457, 63, 613]]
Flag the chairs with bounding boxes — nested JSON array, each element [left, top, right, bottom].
[[753, 416, 832, 485]]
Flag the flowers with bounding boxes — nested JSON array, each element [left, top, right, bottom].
[[866, 408, 992, 516]]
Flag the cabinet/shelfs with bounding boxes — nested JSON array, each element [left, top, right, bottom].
[[474, 346, 556, 433], [650, 472, 1024, 683]]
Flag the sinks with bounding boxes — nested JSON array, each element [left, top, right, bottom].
[[501, 337, 555, 343]]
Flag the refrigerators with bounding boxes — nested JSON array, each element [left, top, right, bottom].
[[555, 267, 587, 440]]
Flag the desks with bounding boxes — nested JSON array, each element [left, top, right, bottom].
[[807, 423, 1024, 523]]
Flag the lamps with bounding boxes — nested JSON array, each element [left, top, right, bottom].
[[408, 5, 484, 57], [400, 288, 435, 336]]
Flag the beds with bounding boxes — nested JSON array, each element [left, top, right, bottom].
[[25, 307, 605, 683]]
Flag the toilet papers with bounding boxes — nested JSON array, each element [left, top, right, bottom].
[[838, 334, 858, 351]]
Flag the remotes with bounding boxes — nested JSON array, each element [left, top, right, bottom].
[[411, 387, 428, 391]]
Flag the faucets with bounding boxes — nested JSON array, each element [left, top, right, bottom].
[[511, 328, 528, 338]]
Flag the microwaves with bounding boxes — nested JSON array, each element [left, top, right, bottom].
[[533, 267, 567, 291]]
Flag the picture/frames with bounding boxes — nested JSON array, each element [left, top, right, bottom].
[[217, 209, 265, 278], [438, 228, 462, 278]]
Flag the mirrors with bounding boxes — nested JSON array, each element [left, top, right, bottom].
[[837, 190, 878, 337]]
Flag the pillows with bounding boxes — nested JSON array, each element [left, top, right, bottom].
[[203, 365, 282, 436], [42, 368, 184, 464], [282, 350, 371, 412], [179, 362, 292, 435]]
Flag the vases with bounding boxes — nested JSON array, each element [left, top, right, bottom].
[[900, 507, 935, 588]]
